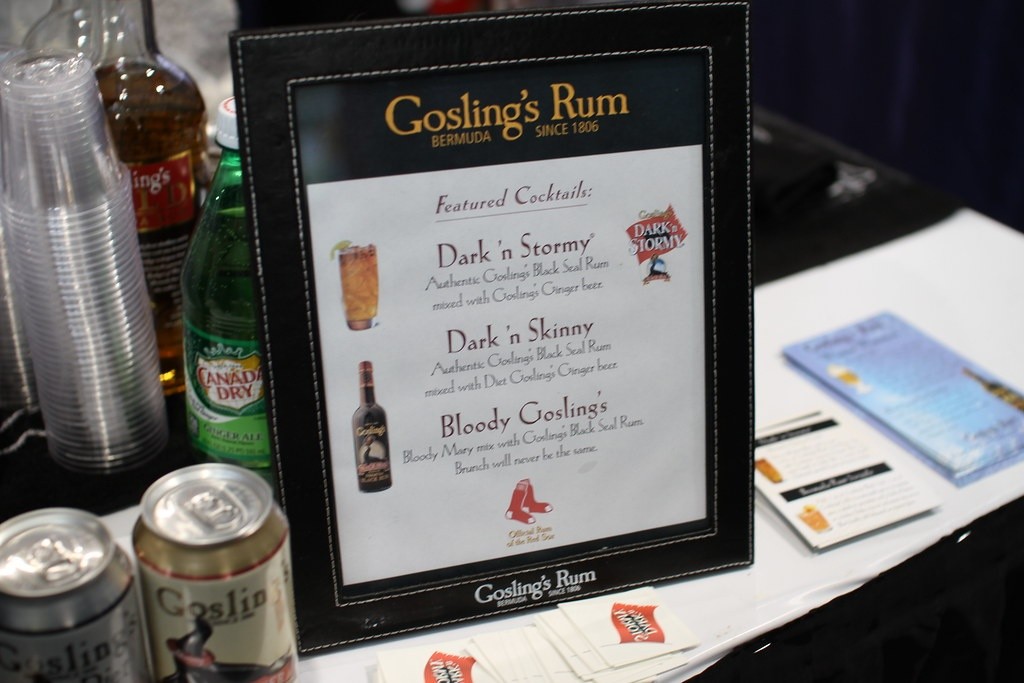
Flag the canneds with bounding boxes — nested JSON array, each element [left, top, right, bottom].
[[131, 460, 299, 683], [0, 508, 160, 683]]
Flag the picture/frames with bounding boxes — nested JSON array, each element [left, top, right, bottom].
[[229, 0, 750, 654]]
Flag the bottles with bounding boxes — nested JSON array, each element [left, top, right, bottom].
[[181, 94, 276, 480], [89, 0, 207, 411]]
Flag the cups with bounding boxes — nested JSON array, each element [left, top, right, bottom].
[[0, 40, 168, 474]]
